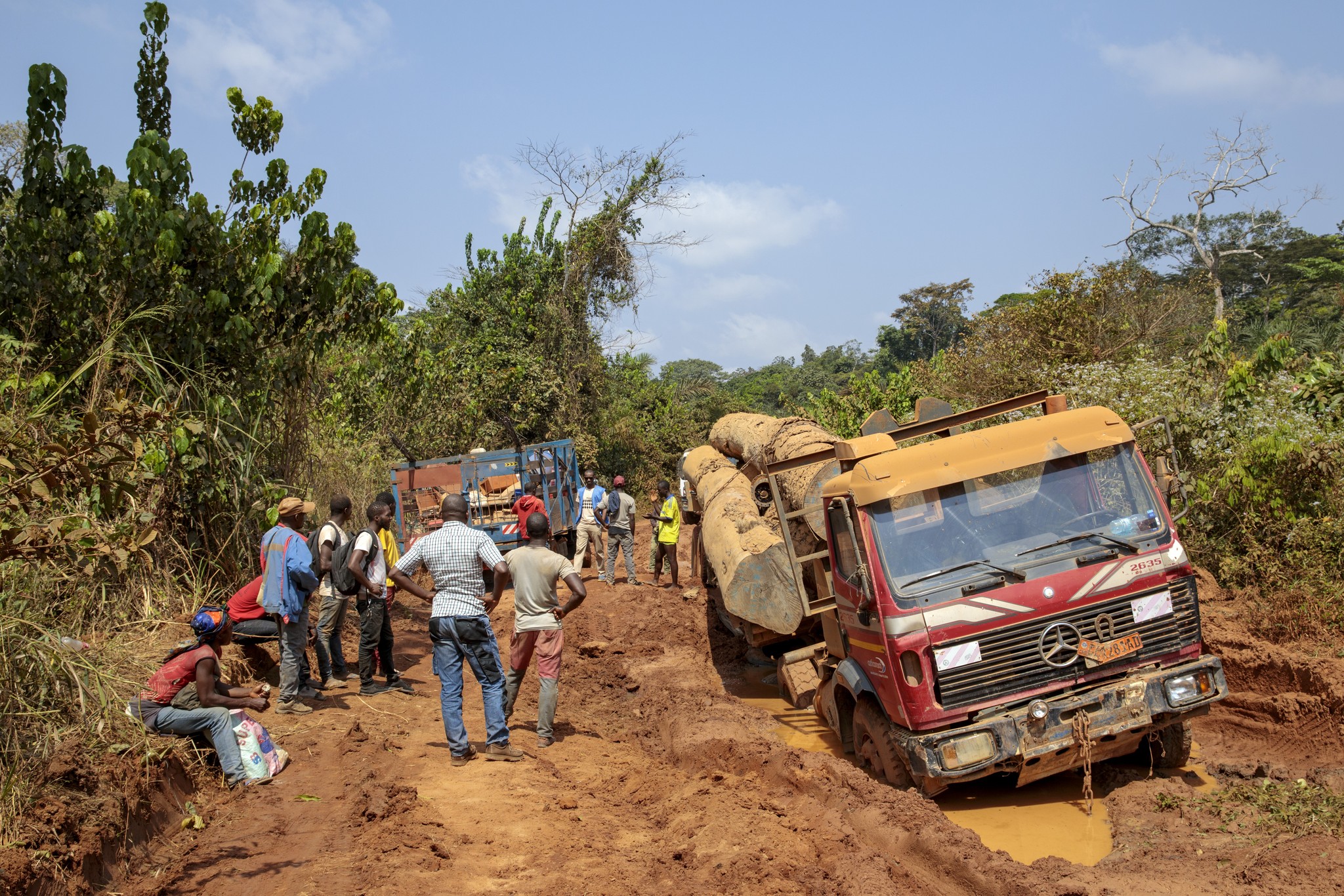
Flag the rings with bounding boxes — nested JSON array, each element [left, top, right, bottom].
[[262, 710, 265, 711], [381, 586, 382, 589]]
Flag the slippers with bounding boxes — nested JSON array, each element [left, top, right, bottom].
[[298, 685, 326, 701], [343, 674, 361, 682], [245, 776, 274, 785], [327, 680, 348, 690], [302, 677, 328, 689], [375, 669, 405, 678]]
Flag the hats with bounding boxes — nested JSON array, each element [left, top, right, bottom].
[[614, 475, 625, 486], [277, 497, 316, 517]]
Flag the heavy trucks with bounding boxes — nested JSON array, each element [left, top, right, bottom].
[[682, 385, 1229, 800], [389, 438, 583, 586]]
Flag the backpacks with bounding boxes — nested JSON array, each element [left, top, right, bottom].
[[331, 528, 379, 595], [305, 522, 341, 588]]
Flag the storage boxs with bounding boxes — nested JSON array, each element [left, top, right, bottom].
[[479, 475, 519, 496], [493, 509, 519, 522]]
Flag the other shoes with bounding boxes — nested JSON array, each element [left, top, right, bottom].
[[634, 582, 644, 585], [649, 571, 655, 574], [556, 584, 563, 589], [537, 736, 557, 748]]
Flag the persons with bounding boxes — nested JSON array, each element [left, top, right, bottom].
[[502, 513, 586, 748], [348, 502, 414, 696], [641, 480, 680, 591], [129, 603, 274, 792], [388, 494, 525, 766], [226, 492, 328, 715], [371, 492, 404, 678], [573, 469, 607, 582], [511, 482, 564, 589], [316, 494, 360, 690], [594, 475, 643, 586], [649, 497, 671, 575]]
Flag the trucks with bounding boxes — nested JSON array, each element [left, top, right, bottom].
[[679, 448, 737, 524]]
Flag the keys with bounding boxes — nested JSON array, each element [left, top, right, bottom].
[[366, 608, 372, 619]]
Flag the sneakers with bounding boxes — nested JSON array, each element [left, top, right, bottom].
[[384, 679, 415, 692], [451, 743, 478, 766], [485, 739, 524, 761], [275, 696, 313, 715], [598, 575, 606, 581], [359, 684, 389, 696]]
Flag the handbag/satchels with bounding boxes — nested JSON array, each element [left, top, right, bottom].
[[255, 582, 266, 608], [170, 643, 221, 711]]
